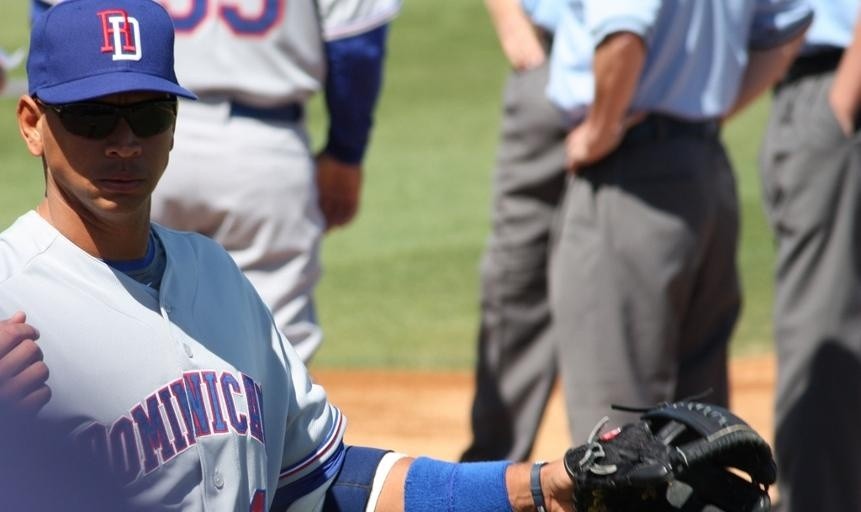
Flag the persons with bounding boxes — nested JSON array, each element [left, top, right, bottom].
[[757, 0, 861, 512], [0, 0, 778, 512], [522, 0, 814, 456], [454, 0, 568, 462], [150, 0, 398, 368]]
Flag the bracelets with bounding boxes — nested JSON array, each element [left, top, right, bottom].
[[403, 455, 514, 512], [530, 461, 549, 512]]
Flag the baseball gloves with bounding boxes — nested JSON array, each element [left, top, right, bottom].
[[565, 399, 777, 511]]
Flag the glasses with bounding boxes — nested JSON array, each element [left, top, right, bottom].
[[35, 95, 178, 140]]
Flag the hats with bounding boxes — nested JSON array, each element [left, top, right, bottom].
[[26, 1, 199, 106]]
